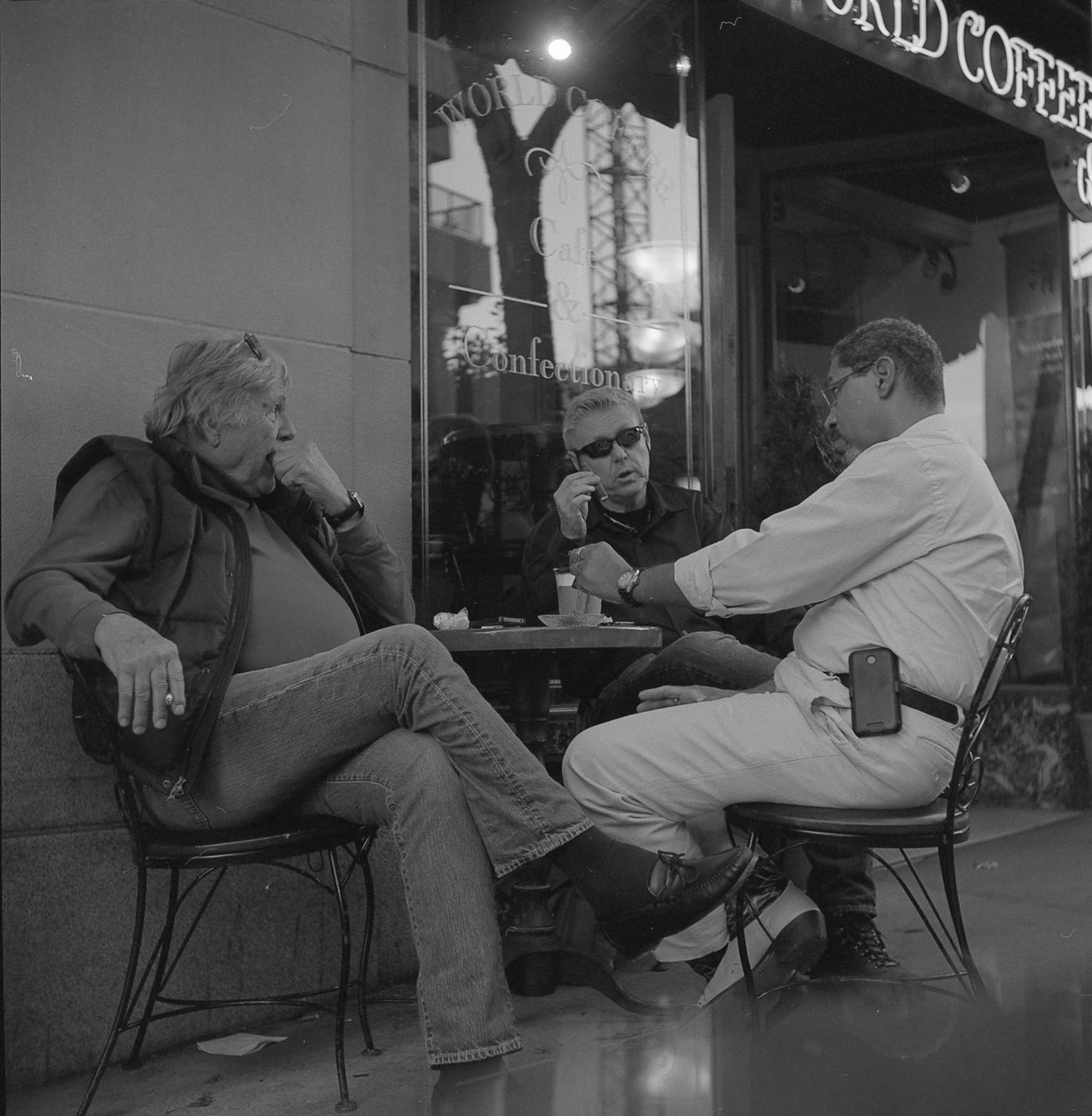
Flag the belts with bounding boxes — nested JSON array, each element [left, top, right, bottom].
[[824, 668, 967, 730]]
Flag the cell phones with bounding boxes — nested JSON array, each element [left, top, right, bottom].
[[848, 648, 902, 738]]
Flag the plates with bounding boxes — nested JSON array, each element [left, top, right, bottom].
[[538, 614, 605, 627]]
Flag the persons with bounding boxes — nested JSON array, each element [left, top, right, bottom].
[[568, 320, 1025, 1043], [5, 333, 761, 1069], [522, 388, 918, 984]]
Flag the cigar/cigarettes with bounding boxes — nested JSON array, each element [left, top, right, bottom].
[[165, 692, 172, 706], [582, 462, 608, 501]]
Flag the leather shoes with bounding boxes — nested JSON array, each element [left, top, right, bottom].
[[595, 846, 756, 959], [677, 882, 832, 1038], [428, 1079, 541, 1116]]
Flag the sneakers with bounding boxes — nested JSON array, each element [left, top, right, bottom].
[[814, 913, 924, 1001]]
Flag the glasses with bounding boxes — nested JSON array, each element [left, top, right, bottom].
[[576, 426, 644, 458], [225, 333, 263, 361], [824, 362, 873, 404]]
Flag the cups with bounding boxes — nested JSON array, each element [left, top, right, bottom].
[[552, 567, 601, 614]]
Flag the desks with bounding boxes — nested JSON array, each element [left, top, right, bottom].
[[426, 626, 685, 1018]]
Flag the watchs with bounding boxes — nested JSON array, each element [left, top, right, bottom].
[[616, 567, 646, 608], [325, 489, 364, 527]]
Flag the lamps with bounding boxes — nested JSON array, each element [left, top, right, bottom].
[[942, 165, 970, 194]]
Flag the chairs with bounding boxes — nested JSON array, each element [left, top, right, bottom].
[[720, 593, 1035, 1025], [57, 645, 383, 1115]]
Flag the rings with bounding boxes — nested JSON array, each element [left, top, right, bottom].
[[674, 698, 678, 705], [573, 562, 580, 573], [578, 547, 585, 556]]
